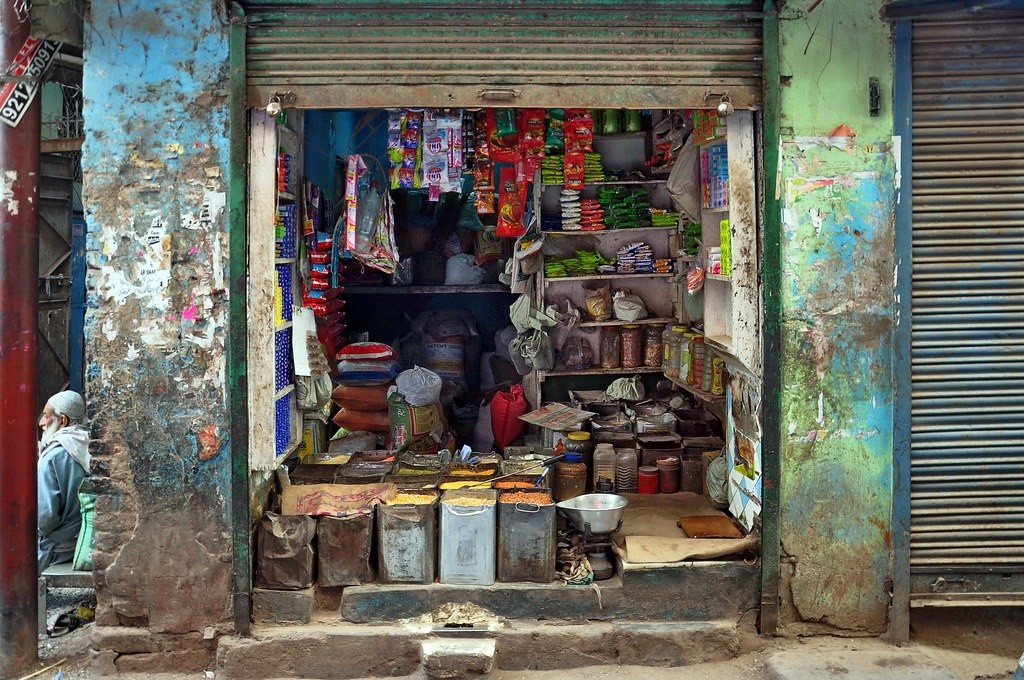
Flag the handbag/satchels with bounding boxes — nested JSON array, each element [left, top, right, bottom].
[[606, 374, 645, 401], [395, 365, 442, 407], [472, 398, 495, 452], [705, 446, 728, 509], [499, 199, 555, 376], [490, 383, 527, 451]]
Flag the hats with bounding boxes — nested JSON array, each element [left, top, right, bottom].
[[48, 390, 85, 419]]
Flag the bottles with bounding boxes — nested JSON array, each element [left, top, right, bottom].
[[593, 444, 616, 493], [638, 466, 659, 495], [616, 448, 638, 494], [553, 453, 586, 502], [564, 432, 594, 492]]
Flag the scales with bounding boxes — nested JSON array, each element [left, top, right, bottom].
[[556, 493, 629, 580]]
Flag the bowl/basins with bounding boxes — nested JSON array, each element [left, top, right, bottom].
[[555, 494, 629, 532]]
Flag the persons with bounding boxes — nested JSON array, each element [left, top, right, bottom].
[[37, 390, 90, 580]]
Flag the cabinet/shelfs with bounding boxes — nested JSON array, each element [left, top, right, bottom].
[[246, 105, 759, 500]]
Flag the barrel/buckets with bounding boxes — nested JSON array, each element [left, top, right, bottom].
[[255, 446, 556, 591], [656, 455, 680, 493], [424, 342, 465, 378]]
